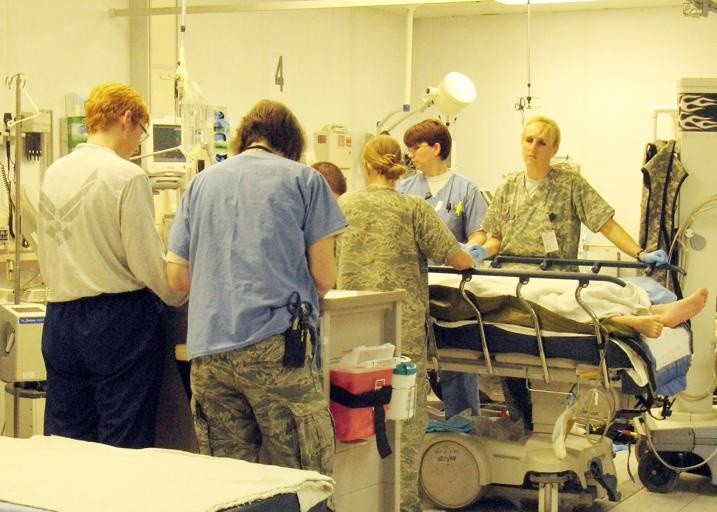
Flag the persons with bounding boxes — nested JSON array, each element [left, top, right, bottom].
[[308, 161, 347, 199], [333, 133, 477, 511], [162, 99, 353, 512], [609, 286, 711, 340], [465, 116, 670, 275], [36, 82, 189, 449], [394, 119, 487, 252]]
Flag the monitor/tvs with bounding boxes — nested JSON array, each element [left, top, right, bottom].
[[141, 116, 188, 173]]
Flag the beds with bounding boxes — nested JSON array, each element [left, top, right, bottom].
[[419, 255, 692, 512]]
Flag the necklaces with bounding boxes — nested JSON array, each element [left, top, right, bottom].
[[245, 145, 272, 153]]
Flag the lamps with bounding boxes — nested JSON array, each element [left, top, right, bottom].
[[378, 71, 477, 132]]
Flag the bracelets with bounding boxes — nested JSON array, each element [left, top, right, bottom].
[[636, 249, 644, 262]]
